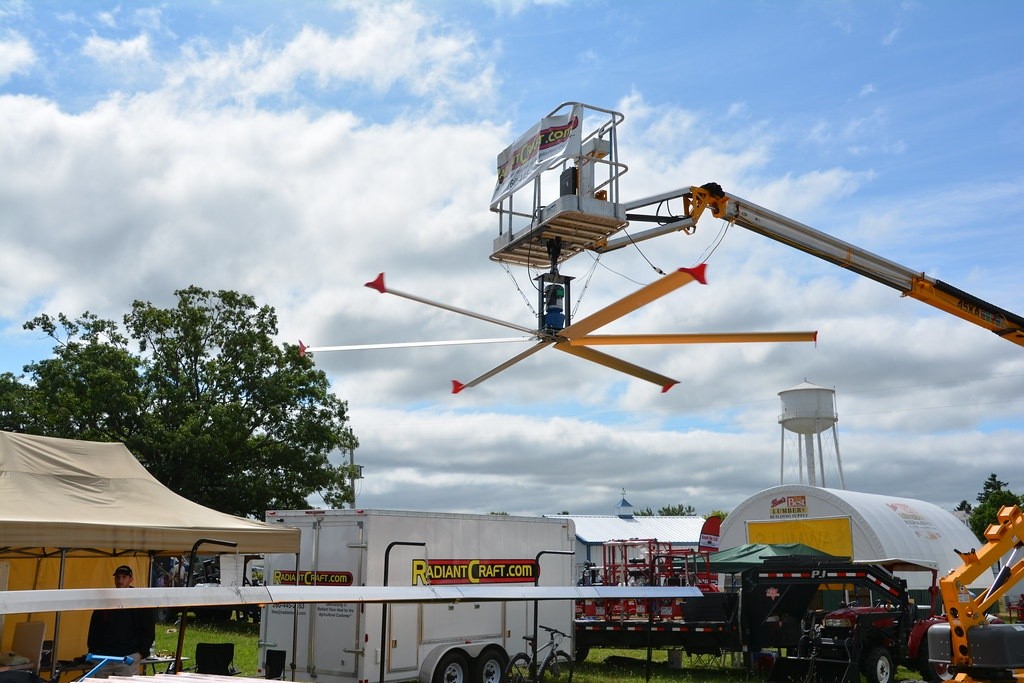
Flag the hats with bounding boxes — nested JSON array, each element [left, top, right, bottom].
[[113, 566, 133, 577]]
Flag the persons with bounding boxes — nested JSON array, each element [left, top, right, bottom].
[[157, 558, 185, 587], [663, 570, 695, 587], [87, 566, 157, 680]]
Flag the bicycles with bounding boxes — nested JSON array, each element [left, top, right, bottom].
[[503, 624, 577, 683]]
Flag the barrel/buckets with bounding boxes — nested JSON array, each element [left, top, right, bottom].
[[668, 651, 682, 668]]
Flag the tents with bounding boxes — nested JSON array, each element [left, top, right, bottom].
[[0, 430, 301, 682]]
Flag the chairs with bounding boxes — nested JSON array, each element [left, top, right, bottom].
[[183, 643, 242, 677], [250, 649, 287, 681], [0, 621, 47, 675], [898, 604, 919, 624]]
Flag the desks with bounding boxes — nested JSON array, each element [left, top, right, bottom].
[[40, 655, 189, 683]]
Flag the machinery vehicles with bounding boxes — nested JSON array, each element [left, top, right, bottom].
[[487, 100, 1024, 683]]
